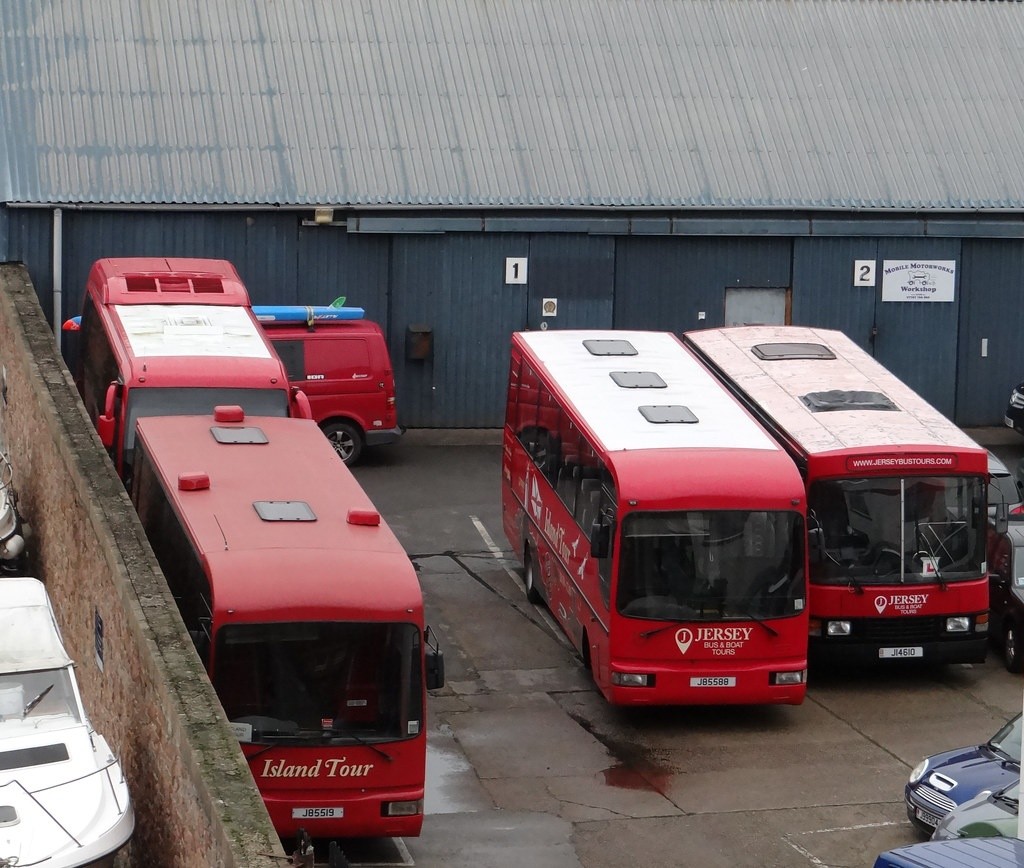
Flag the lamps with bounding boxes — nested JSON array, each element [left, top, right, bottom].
[[315, 208, 334, 224]]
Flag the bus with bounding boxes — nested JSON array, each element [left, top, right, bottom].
[[76, 256, 312, 498], [682, 326, 1009, 674], [129, 406, 445, 837], [504, 330, 828, 707]]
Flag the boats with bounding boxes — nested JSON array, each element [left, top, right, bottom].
[[0, 452, 32, 572], [0, 576, 137, 868]]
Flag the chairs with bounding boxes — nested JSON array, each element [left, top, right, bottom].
[[554, 442, 602, 530], [331, 648, 381, 723], [218, 656, 259, 713]]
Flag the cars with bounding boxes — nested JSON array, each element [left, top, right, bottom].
[[1004, 382, 1024, 437], [905, 710, 1024, 838]]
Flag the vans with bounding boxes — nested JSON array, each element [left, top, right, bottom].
[[988, 517, 1024, 673], [267, 321, 406, 469], [946, 449, 1024, 526]]
